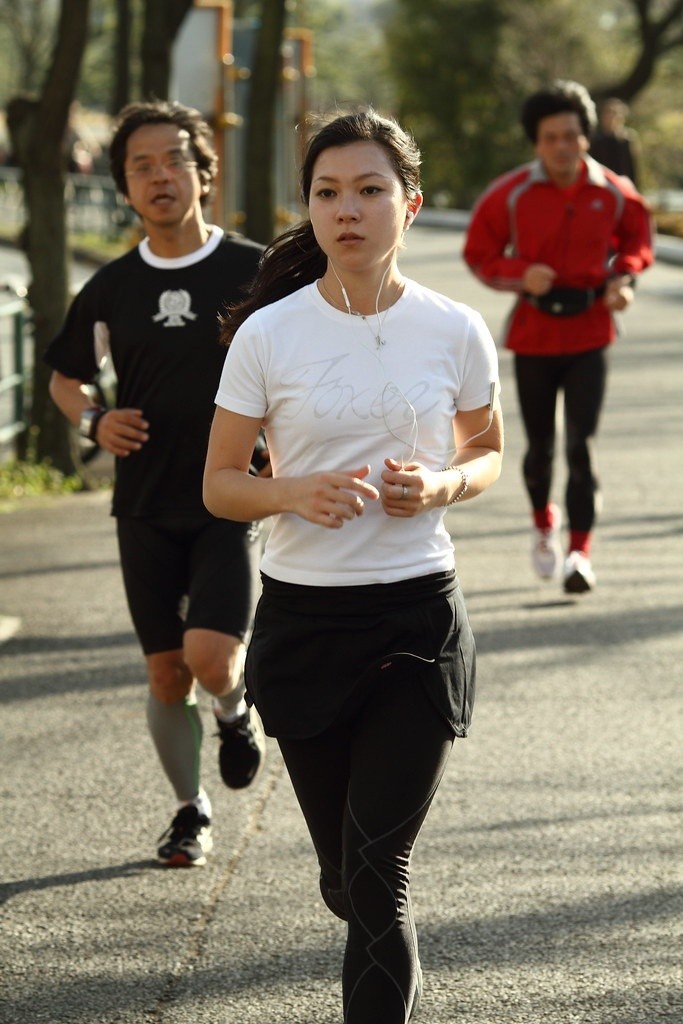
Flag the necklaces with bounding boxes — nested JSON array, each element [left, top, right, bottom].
[[322, 277, 403, 344]]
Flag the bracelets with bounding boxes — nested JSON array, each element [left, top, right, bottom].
[[437, 465, 470, 508]]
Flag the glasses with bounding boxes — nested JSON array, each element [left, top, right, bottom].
[[125, 158, 198, 178]]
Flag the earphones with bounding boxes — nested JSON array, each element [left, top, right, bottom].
[[406, 211, 413, 230]]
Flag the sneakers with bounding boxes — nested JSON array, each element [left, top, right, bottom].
[[531, 503, 563, 580], [212, 696, 265, 789], [154, 804, 213, 866], [564, 550, 595, 593]]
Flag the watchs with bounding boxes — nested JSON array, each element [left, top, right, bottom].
[[79, 406, 109, 442]]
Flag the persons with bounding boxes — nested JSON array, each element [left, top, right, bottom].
[[45, 102, 273, 869], [202, 110, 503, 1024], [463, 79, 656, 593], [591, 99, 638, 288]]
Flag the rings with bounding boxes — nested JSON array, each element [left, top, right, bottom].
[[400, 486, 407, 499]]
